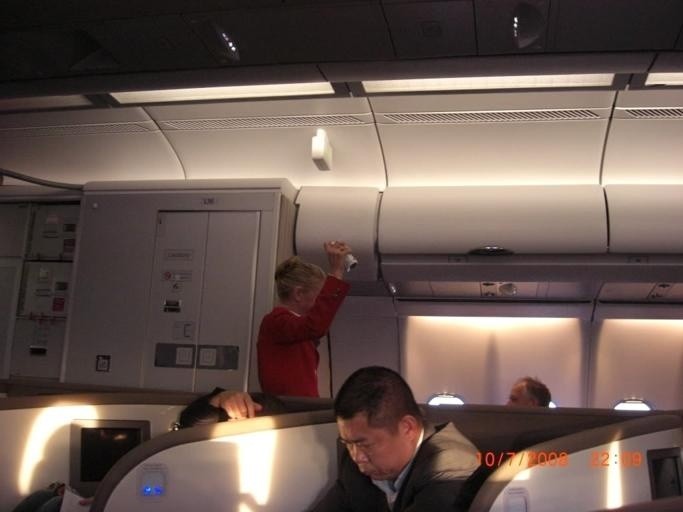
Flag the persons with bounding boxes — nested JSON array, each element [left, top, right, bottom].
[[507, 377, 551, 407], [258, 239, 359, 399], [171, 386, 262, 430], [314, 365, 487, 511]]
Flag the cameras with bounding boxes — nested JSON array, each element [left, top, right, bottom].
[[331, 241, 358, 273]]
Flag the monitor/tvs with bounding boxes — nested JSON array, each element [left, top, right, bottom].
[[69, 419, 150, 498]]
